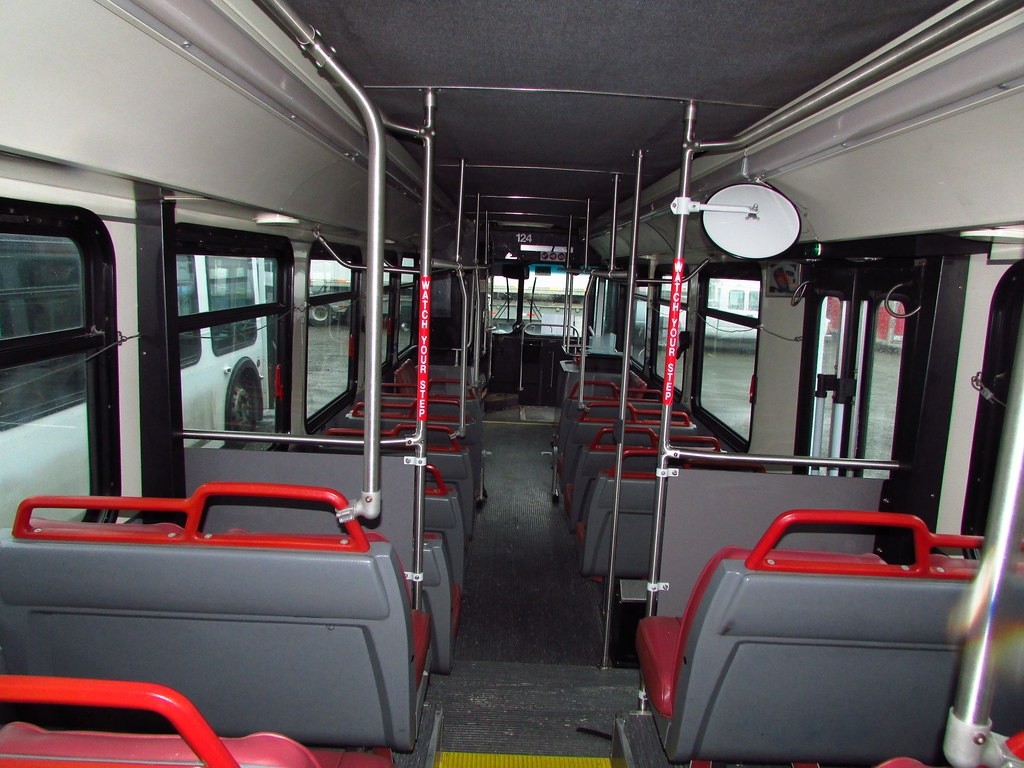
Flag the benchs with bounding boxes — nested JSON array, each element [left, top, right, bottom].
[[1, 482, 435, 751]]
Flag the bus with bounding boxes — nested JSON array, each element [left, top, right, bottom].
[[0, 225, 279, 544], [252, 260, 760, 341]]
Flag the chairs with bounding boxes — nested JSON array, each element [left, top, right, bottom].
[[322, 360, 486, 675], [637, 510, 985, 764], [555, 381, 727, 576]]
